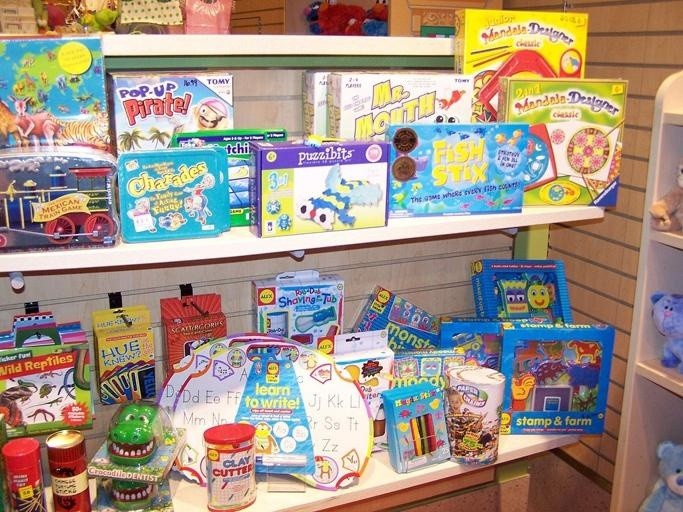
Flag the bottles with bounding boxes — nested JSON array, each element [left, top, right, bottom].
[[202, 422, 259, 512], [45, 428, 93, 512], [1, 437, 46, 512]]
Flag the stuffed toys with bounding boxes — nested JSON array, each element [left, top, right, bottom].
[[303, 1, 389, 36], [636, 440, 682, 511], [651, 291, 683, 372], [648, 160, 682, 232], [33, 1, 118, 35]]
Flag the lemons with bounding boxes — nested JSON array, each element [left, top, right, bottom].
[[79, 10, 116, 31]]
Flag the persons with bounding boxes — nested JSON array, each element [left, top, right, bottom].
[[447, 390, 470, 424]]
